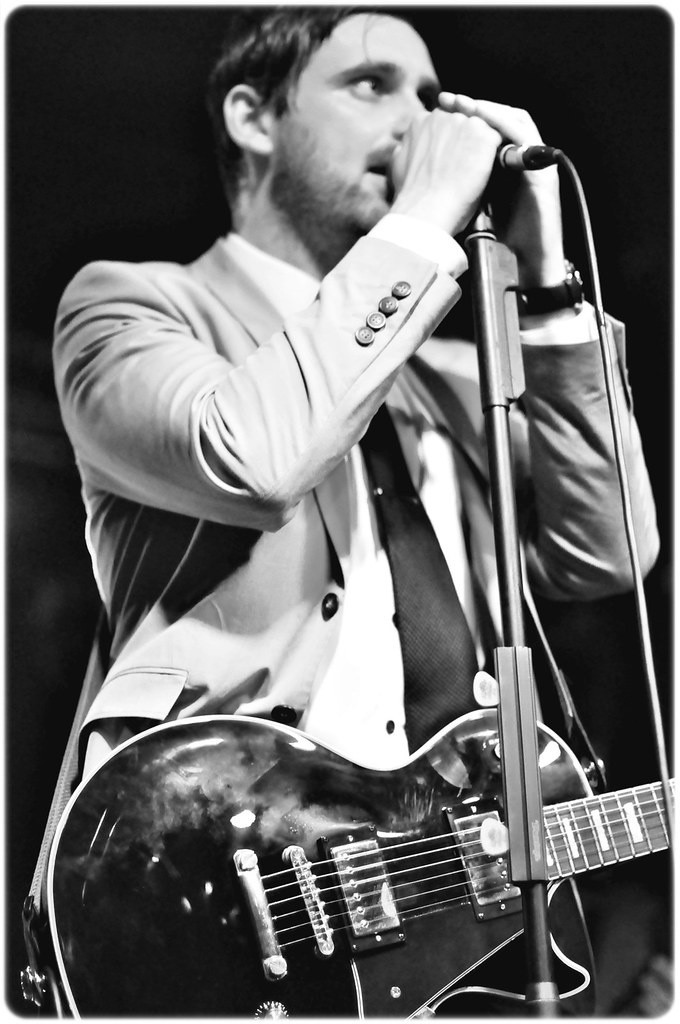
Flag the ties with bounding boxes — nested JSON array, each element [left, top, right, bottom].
[[358, 401, 482, 755]]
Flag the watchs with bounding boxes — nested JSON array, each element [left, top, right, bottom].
[[516, 258, 585, 317]]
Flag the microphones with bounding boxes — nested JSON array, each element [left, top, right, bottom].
[[489, 144, 567, 191]]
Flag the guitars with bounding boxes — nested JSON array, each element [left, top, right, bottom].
[[36, 701, 674, 1018]]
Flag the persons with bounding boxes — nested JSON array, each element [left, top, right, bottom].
[[52, 6, 660, 1017]]
